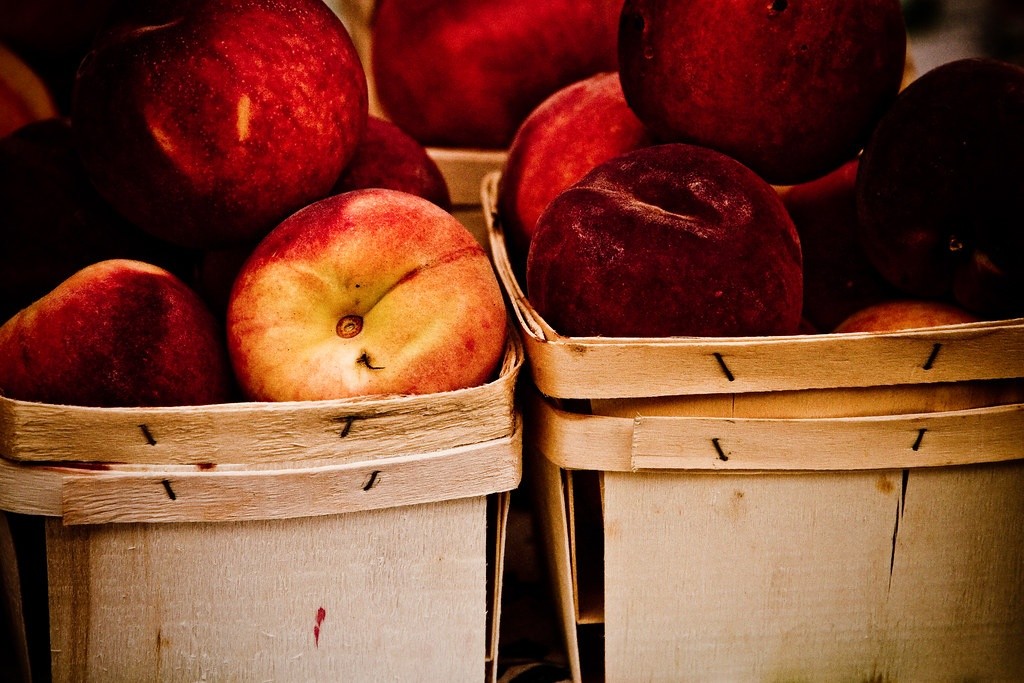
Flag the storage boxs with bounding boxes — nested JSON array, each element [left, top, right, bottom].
[[0, 167, 1023, 683]]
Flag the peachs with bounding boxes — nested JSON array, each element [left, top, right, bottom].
[[0, 0, 630, 408], [500, 1, 1024, 348]]
